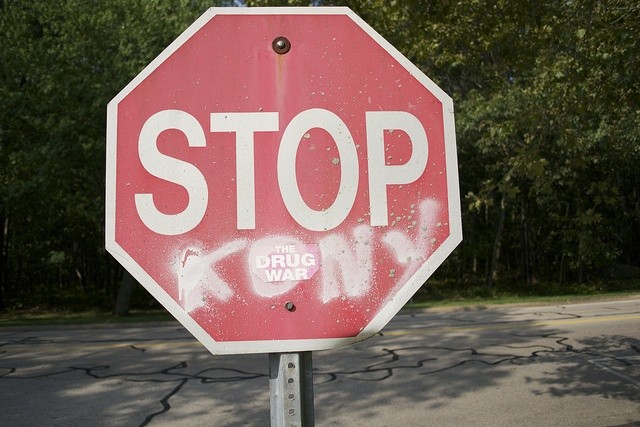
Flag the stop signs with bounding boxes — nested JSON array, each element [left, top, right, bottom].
[[102, 4, 465, 357]]
